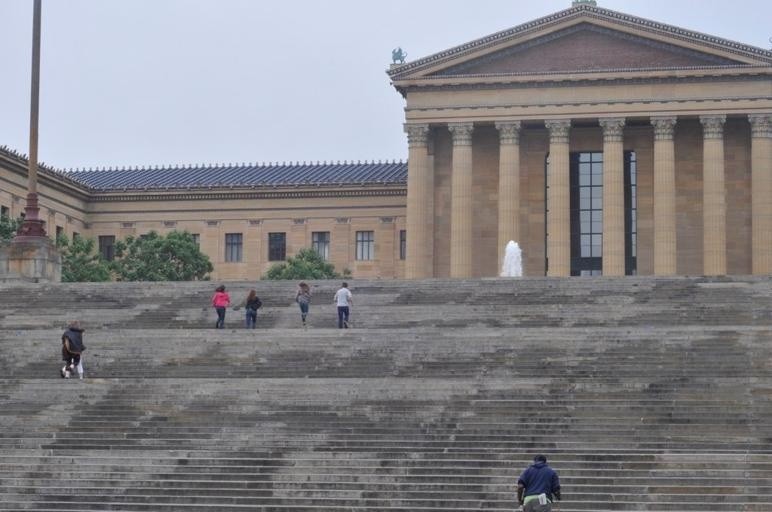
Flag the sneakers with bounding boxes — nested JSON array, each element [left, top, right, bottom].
[[60, 369, 83, 380]]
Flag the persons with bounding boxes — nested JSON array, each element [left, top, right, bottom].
[[517, 455, 561, 512], [212, 285, 231, 329], [61, 320, 87, 378], [334, 282, 353, 328], [245, 290, 262, 329], [296, 281, 312, 326]]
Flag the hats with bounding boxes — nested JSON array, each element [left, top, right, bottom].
[[68, 320, 81, 330]]
